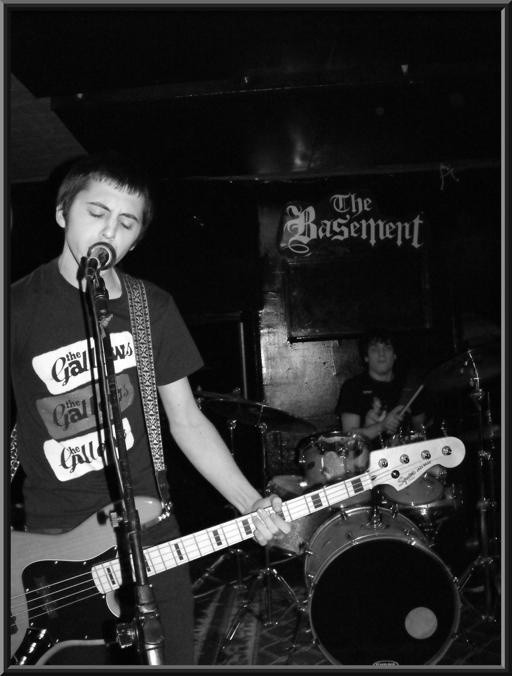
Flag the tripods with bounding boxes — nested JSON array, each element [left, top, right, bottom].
[[194, 420, 255, 599], [453, 391, 502, 629], [218, 421, 314, 661]]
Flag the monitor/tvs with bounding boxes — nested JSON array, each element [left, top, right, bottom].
[[280, 253, 430, 343]]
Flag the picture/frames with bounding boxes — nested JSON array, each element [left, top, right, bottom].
[[278, 175, 500, 345]]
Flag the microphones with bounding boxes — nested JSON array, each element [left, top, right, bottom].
[[82, 242, 115, 277]]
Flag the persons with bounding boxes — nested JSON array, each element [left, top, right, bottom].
[[332, 324, 428, 441], [4, 148, 293, 669]]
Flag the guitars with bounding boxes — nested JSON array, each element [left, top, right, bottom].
[[11, 437, 467, 665]]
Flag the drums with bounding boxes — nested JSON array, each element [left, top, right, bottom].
[[378, 432, 448, 504], [304, 503, 462, 664], [299, 431, 370, 485], [264, 474, 340, 559], [379, 482, 466, 584]]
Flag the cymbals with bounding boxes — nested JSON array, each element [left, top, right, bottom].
[[192, 391, 271, 407], [458, 424, 499, 440], [195, 399, 317, 434], [422, 346, 501, 395]]
